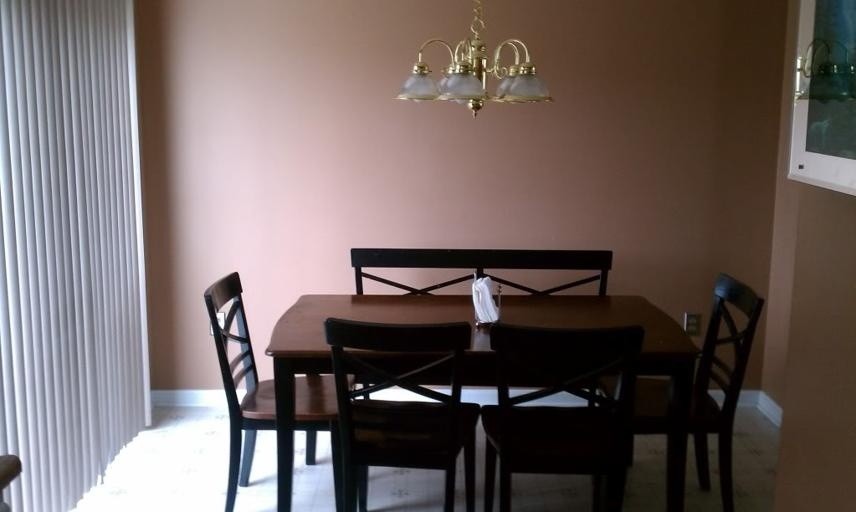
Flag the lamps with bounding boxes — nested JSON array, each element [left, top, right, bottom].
[[394, 0, 553, 119]]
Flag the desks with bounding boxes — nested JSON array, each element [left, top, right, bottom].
[[264, 293, 703, 512]]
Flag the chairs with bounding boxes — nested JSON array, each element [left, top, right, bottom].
[[596, 271, 765, 511], [350, 248, 614, 301], [324, 316, 482, 510], [481, 323, 643, 511], [203, 268, 343, 512]]
